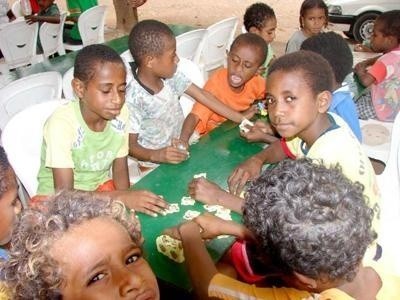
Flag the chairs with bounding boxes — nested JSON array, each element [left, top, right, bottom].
[[0, 0, 400, 299]]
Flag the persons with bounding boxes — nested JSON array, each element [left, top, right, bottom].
[[353, 10, 400, 123], [120, 18, 259, 183], [37, 44, 170, 218], [243, 1, 277, 77], [0, 189, 160, 299], [284, 0, 328, 55], [64, 0, 99, 45], [169, 34, 268, 152], [24, 0, 60, 54], [0, 144, 24, 299], [238, 30, 364, 148], [163, 157, 400, 300], [187, 49, 381, 287]]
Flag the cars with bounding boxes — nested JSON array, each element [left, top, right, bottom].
[[321, 0, 400, 47]]
